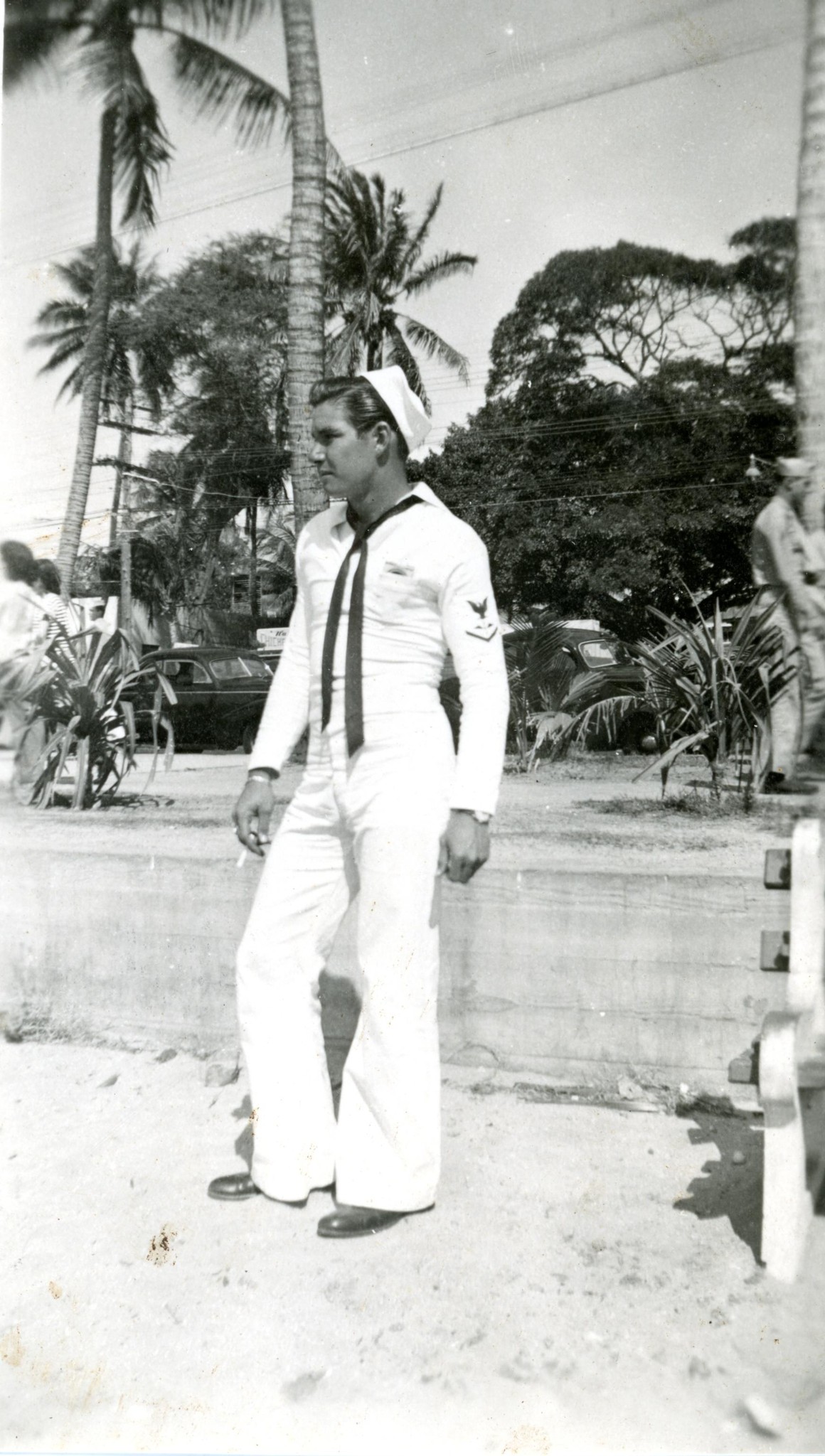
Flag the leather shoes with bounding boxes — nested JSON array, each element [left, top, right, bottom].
[[208, 1172, 262, 1200], [317, 1202, 434, 1238], [760, 779, 818, 795]]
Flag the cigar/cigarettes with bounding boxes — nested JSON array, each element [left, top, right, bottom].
[[237, 834, 255, 867]]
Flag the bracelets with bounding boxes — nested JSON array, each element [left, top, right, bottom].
[[244, 776, 270, 786]]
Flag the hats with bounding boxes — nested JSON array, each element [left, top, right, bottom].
[[778, 457, 817, 476], [361, 364, 432, 455]]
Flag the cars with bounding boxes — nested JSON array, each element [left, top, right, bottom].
[[500, 626, 647, 747], [107, 647, 275, 754]]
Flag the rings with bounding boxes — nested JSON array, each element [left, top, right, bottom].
[[233, 826, 239, 835]]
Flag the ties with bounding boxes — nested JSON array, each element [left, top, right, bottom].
[[321, 495, 425, 758]]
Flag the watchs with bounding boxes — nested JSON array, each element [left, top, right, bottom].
[[458, 809, 490, 824]]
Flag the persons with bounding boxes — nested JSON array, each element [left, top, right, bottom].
[[1, 541, 49, 805], [26, 559, 77, 685], [87, 598, 112, 677], [748, 457, 825, 794], [209, 363, 512, 1237]]
